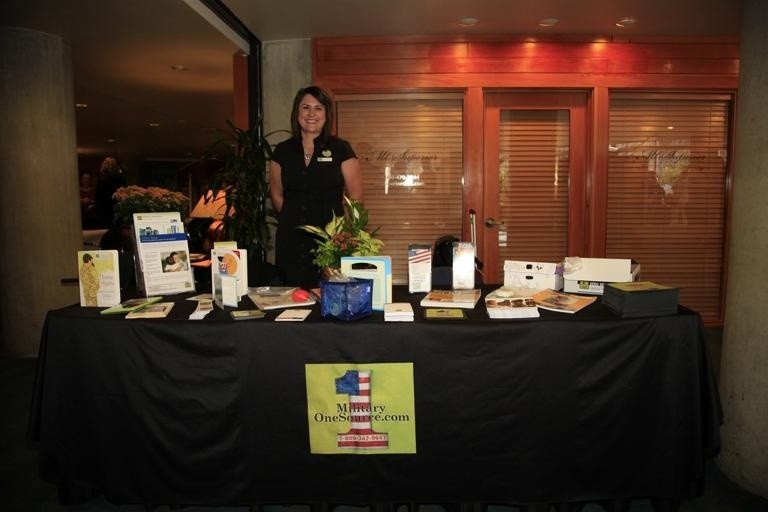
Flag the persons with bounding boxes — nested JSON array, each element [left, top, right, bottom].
[[269, 87, 365, 286], [79, 173, 96, 203], [88, 263, 99, 284], [93, 157, 126, 219], [80, 252, 100, 307]]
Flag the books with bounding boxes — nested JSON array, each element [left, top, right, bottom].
[[128, 209, 194, 295], [382, 282, 681, 322], [99, 240, 320, 322], [77, 249, 123, 307]]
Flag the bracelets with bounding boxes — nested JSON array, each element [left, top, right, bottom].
[[302, 142, 313, 160]]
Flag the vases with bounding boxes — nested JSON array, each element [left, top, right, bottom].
[[100, 228, 135, 290]]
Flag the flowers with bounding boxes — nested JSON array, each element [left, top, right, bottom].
[[298, 196, 387, 282], [108, 179, 193, 237]]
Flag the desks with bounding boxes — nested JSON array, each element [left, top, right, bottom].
[[25, 284, 733, 510]]
